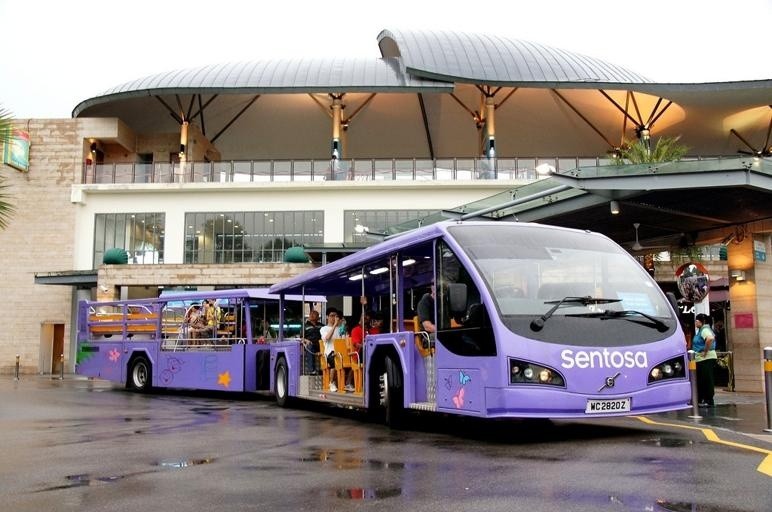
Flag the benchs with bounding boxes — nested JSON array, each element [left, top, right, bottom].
[[89, 311, 241, 345]]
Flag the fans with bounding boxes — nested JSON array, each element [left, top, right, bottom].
[[627, 221, 666, 251]]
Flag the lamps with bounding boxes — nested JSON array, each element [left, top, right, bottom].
[[608, 198, 620, 215], [729, 268, 747, 285]]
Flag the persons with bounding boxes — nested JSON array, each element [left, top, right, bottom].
[[180, 295, 384, 394], [693, 312, 720, 412], [417, 273, 464, 350]]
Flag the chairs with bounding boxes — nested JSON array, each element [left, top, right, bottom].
[[302, 313, 438, 396]]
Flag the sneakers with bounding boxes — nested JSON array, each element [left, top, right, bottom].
[[328, 381, 338, 392], [687, 398, 715, 409], [344, 383, 355, 392]]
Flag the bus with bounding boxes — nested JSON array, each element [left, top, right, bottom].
[[75, 288, 329, 392], [265, 220, 691, 417]]
